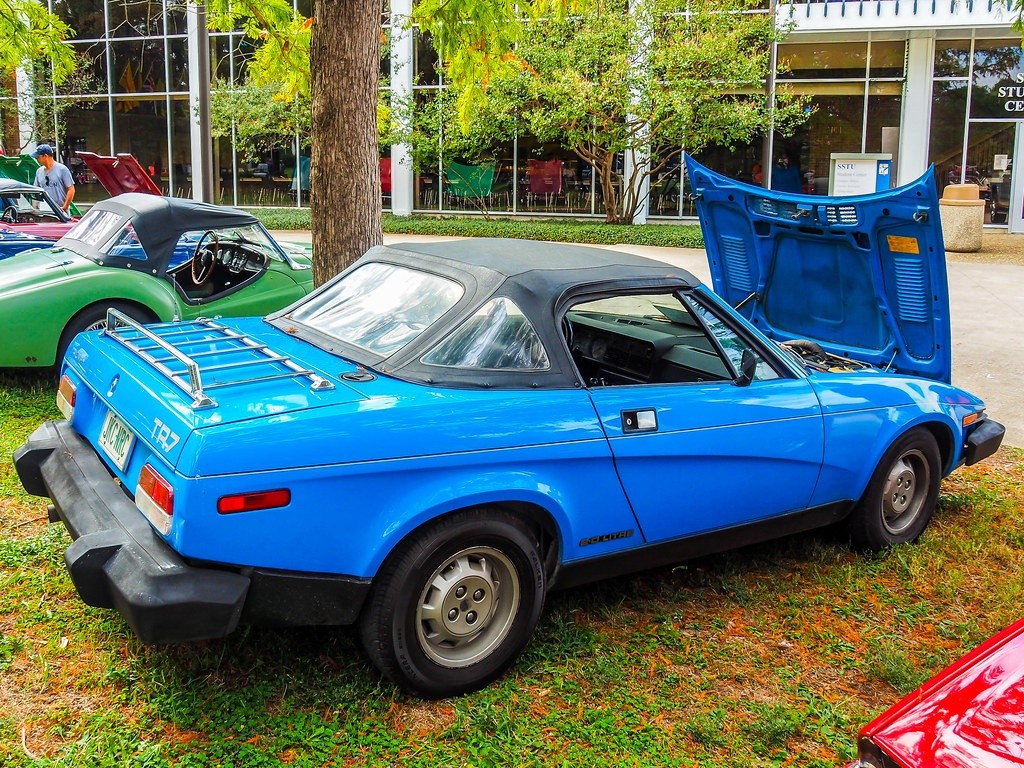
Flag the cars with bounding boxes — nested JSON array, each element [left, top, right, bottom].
[[13, 153, 1005, 700], [0, 193, 314, 378], [0, 150, 164, 260]]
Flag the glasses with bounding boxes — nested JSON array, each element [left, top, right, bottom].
[[45, 176, 49, 186]]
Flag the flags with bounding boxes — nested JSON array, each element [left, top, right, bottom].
[[114, 57, 158, 113]]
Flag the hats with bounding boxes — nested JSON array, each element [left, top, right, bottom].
[[30, 145, 53, 158]]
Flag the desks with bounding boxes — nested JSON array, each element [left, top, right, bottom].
[[240, 177, 292, 183], [160, 176, 192, 181]]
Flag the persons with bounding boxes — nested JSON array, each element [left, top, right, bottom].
[[752, 159, 763, 184], [31, 144, 75, 220], [280, 160, 285, 174]]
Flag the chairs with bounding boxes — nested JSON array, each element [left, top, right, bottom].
[[153, 155, 311, 203], [380, 157, 391, 199], [419, 160, 604, 212]]
